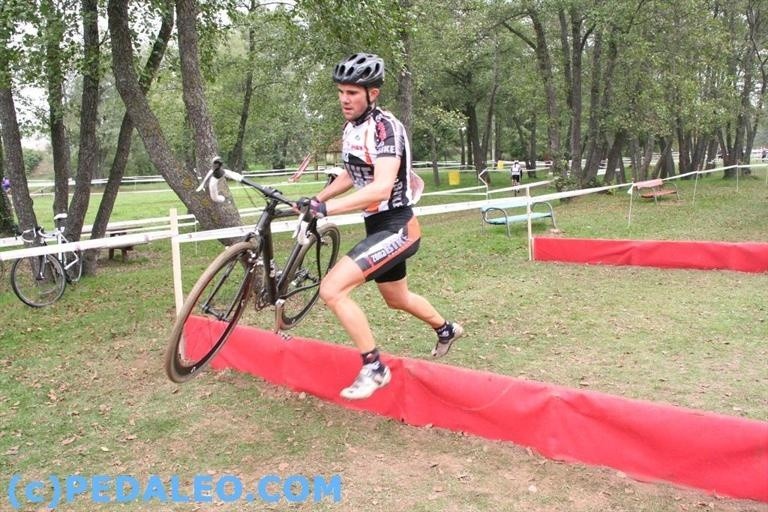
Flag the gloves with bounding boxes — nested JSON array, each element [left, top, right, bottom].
[[297, 196, 328, 223]]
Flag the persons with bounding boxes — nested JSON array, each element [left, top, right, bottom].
[[291, 49, 463, 402], [511, 159, 523, 192]]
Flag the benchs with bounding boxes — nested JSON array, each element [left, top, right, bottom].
[[635, 177, 680, 206], [481, 195, 562, 238]]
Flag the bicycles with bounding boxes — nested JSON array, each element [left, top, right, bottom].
[[164, 155, 344, 384], [11, 213, 83, 308], [512, 174, 522, 196]]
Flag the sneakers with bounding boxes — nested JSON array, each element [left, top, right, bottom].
[[338, 361, 393, 401], [430, 320, 464, 359]]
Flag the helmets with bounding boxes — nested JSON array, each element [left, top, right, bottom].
[[332, 52, 386, 89]]
